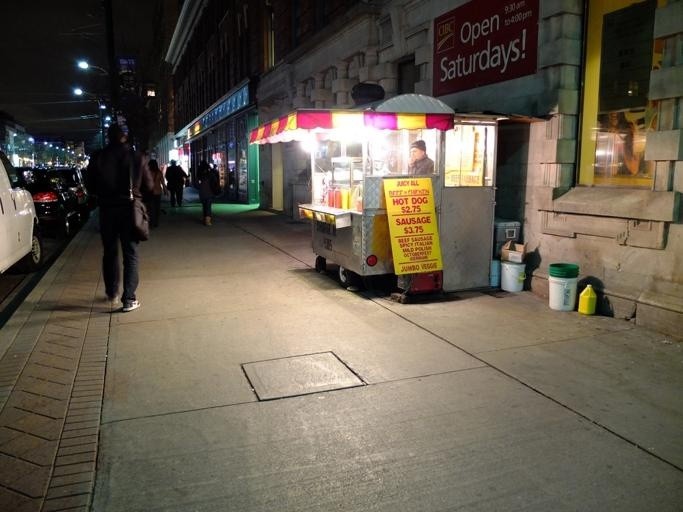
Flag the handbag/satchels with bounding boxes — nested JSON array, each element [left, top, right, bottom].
[[130, 195, 151, 243]]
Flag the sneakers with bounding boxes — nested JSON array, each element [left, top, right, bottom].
[[105, 291, 119, 305], [205, 217, 212, 225], [122, 298, 141, 311], [171, 204, 182, 207]]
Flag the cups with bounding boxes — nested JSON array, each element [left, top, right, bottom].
[[300, 209, 334, 223]]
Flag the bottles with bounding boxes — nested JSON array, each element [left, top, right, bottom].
[[327, 187, 361, 211]]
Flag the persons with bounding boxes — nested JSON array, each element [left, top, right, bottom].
[[164, 160, 187, 207], [147, 156, 169, 231], [80, 120, 153, 312], [407, 140, 434, 177], [193, 158, 216, 227]]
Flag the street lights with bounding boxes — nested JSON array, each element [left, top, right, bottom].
[[75, 88, 105, 149], [81, 60, 110, 75], [8, 133, 89, 167]]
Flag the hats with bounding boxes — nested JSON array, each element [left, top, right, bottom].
[[409, 139, 426, 152]]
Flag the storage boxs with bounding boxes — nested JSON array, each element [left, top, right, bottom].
[[492, 216, 522, 260], [499, 240, 528, 265]]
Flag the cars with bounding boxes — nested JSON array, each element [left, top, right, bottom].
[[212, 165, 246, 186], [0, 149, 98, 276]]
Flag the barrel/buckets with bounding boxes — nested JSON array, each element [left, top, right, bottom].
[[548, 276, 577, 312], [500, 263, 526, 293], [549, 263, 580, 278]]
